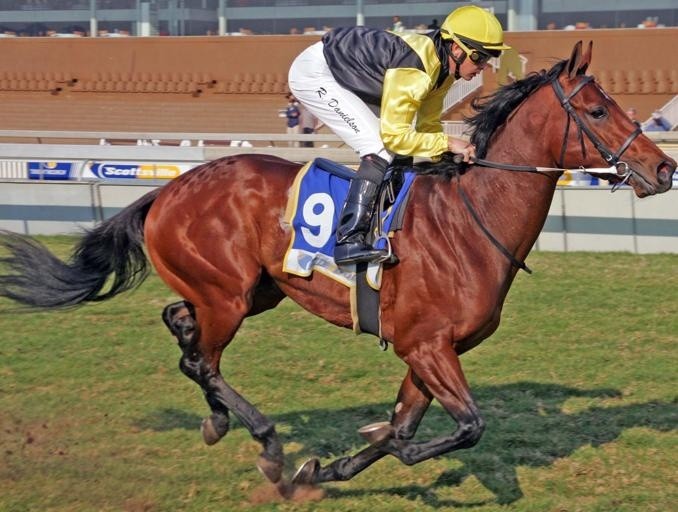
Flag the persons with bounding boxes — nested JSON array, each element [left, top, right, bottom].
[[288, 5, 512, 266], [626, 107, 641, 127], [545, 20, 557, 30], [384, 16, 406, 33], [618, 20, 627, 28], [277, 92, 324, 147]]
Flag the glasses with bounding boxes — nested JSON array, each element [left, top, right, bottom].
[[467, 48, 492, 71]]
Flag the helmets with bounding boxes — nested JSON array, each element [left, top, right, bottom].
[[441, 5, 512, 57]]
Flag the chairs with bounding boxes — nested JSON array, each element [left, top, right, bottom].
[[586, 68, 678, 95], [0, 70, 291, 95]]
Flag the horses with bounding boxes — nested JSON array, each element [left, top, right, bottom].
[[0, 38, 678, 487]]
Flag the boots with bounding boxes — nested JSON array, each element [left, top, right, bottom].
[[333, 153, 399, 266]]
